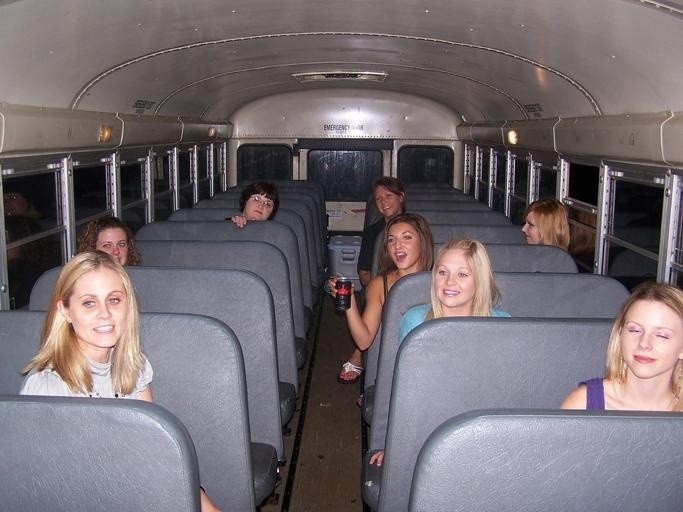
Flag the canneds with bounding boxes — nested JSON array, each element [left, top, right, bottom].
[[335, 278, 350, 311]]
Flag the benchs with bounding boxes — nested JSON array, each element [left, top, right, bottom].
[[362, 183, 682, 512], [0, 181, 328, 512]]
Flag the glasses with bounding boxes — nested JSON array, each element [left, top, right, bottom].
[[251, 196, 272, 209]]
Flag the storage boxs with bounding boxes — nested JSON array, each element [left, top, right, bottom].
[[328, 235, 363, 293]]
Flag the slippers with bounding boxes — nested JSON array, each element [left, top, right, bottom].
[[337, 361, 364, 384]]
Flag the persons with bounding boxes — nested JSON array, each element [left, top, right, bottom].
[[18, 251, 221, 512], [329, 214, 434, 352], [368, 239, 511, 468], [225, 181, 280, 229], [74, 216, 142, 266], [337, 176, 407, 405], [521, 199, 571, 249], [4, 187, 32, 217], [568, 212, 596, 253], [560, 280, 682, 411]]
